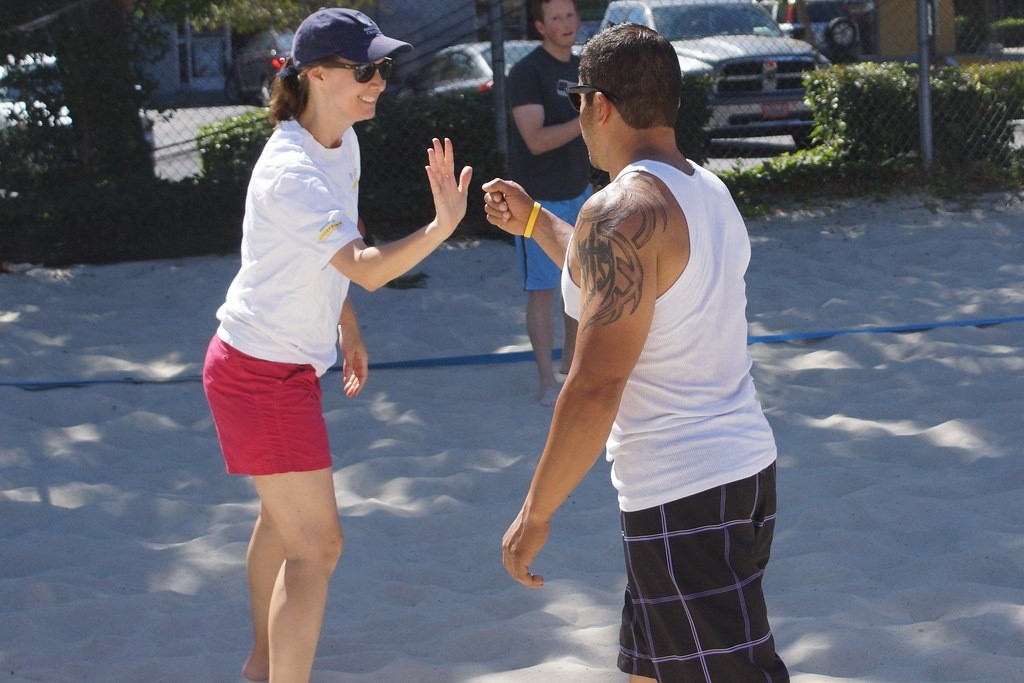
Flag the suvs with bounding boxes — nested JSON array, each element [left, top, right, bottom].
[[598, 1, 835, 149], [758, 0, 860, 64]]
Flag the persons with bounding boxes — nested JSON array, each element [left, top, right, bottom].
[[505, 0, 581, 406], [480, 22, 790, 683], [203, 8, 472, 683]]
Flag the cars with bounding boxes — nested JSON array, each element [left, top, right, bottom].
[[221, 27, 295, 107], [395, 40, 584, 136]]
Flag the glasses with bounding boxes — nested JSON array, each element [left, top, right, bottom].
[[301, 57, 393, 83], [565, 85, 618, 113]]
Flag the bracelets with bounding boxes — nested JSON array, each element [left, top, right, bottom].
[[524, 201, 541, 238]]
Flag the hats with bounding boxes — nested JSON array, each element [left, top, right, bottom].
[[276, 7, 413, 77]]
